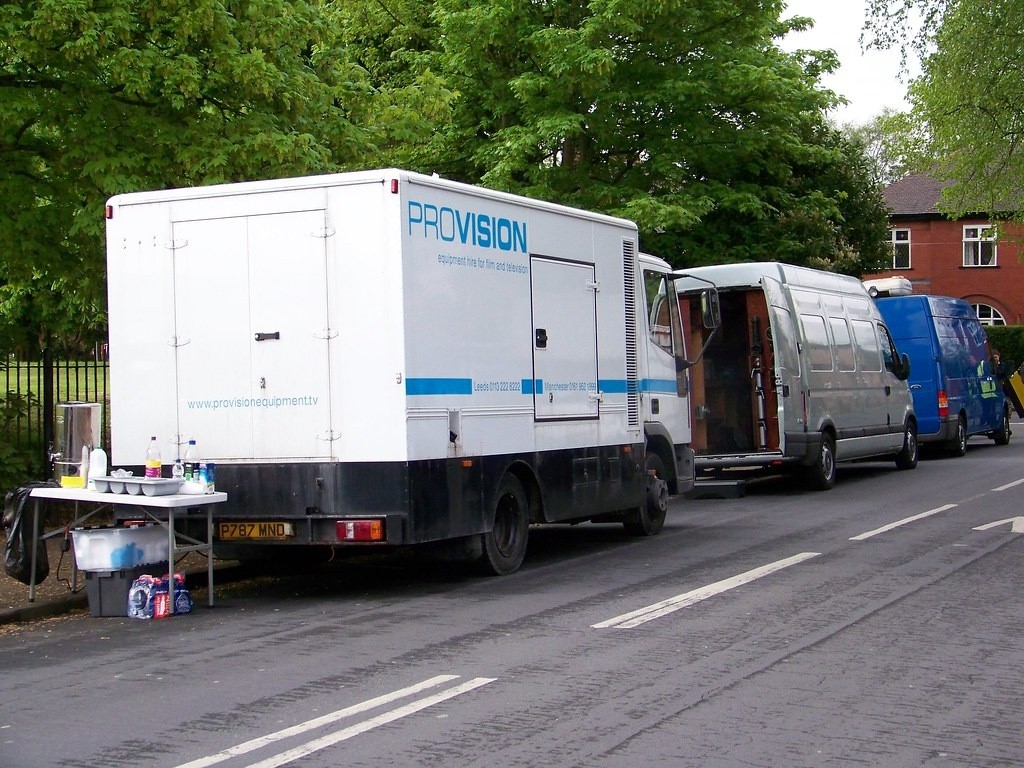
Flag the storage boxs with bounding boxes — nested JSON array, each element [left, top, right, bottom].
[[82, 561, 170, 616], [68, 525, 169, 572]]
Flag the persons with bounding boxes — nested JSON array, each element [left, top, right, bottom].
[[993, 349, 1012, 419]]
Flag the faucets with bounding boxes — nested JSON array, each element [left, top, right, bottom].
[[47, 439, 62, 462]]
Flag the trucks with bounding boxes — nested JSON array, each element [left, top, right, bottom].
[[106, 169, 723, 578]]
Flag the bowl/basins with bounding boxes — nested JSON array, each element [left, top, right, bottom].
[[60, 475, 85, 488]]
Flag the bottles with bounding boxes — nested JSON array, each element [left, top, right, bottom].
[[81, 443, 88, 486], [87, 445, 107, 491], [172, 439, 216, 494], [126, 579, 193, 619], [145, 434, 163, 478]]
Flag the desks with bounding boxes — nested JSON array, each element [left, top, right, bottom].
[[18, 487, 227, 616]]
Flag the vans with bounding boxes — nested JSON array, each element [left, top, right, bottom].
[[649, 262, 920, 490], [861, 276, 1013, 458]]
